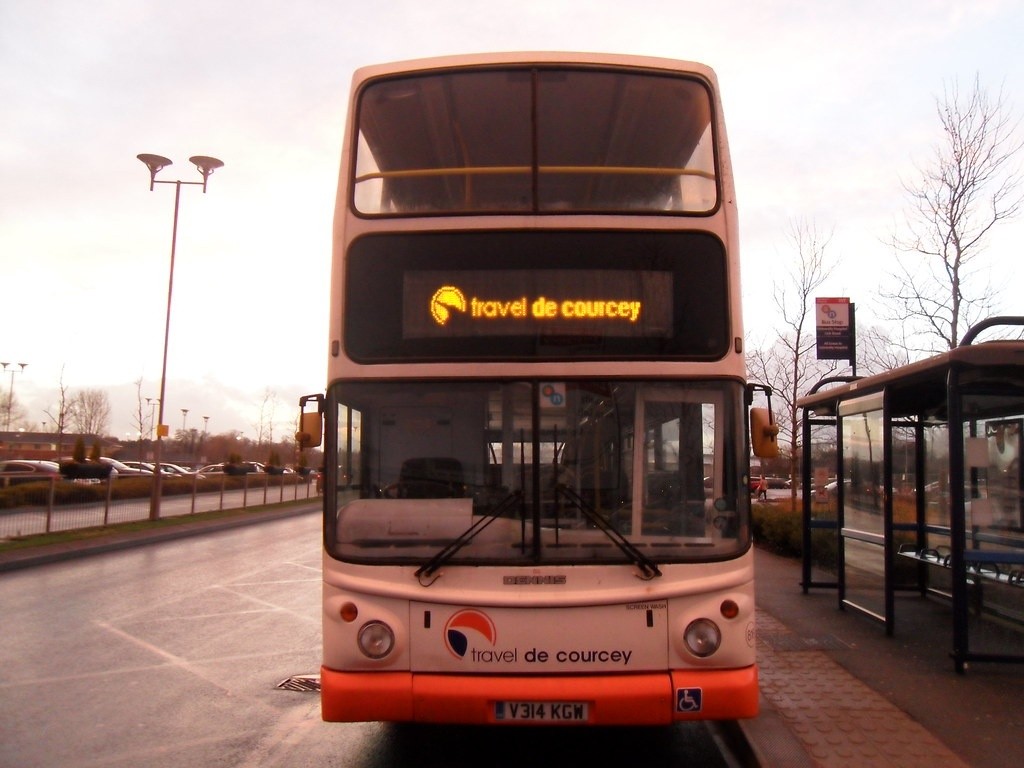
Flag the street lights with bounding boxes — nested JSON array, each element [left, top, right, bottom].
[[137, 154, 226, 518], [1, 362, 29, 432], [202, 416, 209, 436], [146, 398, 159, 440], [181, 408, 190, 432]]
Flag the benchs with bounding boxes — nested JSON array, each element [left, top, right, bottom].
[[897, 543, 1024, 617]]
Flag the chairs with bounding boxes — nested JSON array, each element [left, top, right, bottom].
[[394, 457, 465, 500]]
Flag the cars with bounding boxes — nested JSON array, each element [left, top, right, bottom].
[[0, 456, 321, 493], [703, 474, 959, 507]]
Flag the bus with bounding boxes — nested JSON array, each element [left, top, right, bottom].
[[295, 48, 780, 728]]
